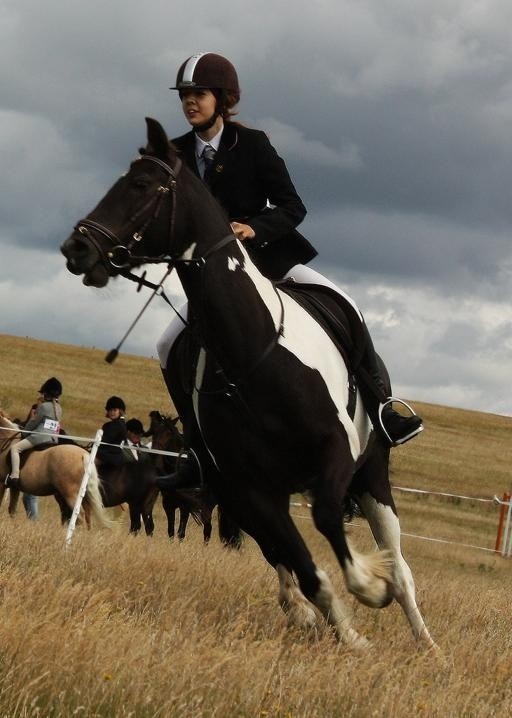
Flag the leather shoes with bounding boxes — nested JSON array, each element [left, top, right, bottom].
[[0, 473, 21, 490]]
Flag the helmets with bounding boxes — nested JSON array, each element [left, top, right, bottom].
[[105, 395, 127, 411], [166, 51, 243, 135], [37, 375, 63, 395], [125, 418, 144, 434]]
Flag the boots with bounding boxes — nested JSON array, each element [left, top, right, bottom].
[[152, 414, 207, 495], [353, 369, 426, 446]]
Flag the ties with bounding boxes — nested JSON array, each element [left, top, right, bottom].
[[199, 143, 219, 195]]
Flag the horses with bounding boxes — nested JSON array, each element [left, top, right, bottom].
[[0, 409, 118, 533], [58, 426, 161, 537], [56, 114, 444, 662], [148, 409, 234, 551]]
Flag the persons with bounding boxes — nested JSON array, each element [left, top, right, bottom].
[[10, 378, 64, 490], [94, 398, 125, 451], [8, 403, 41, 525], [114, 417, 150, 479], [156, 53, 425, 494]]
